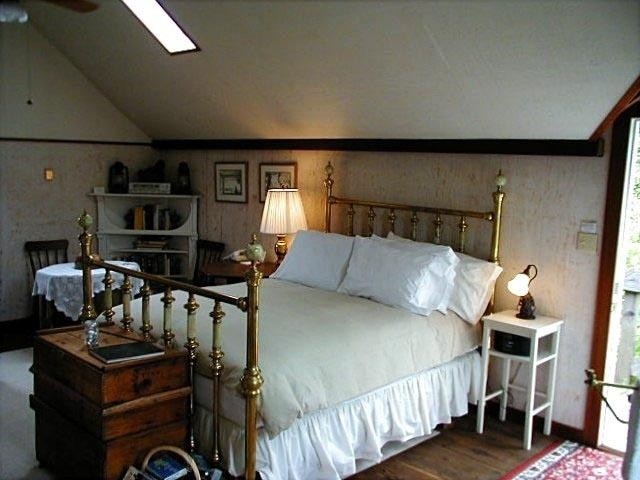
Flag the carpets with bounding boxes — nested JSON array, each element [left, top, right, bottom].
[[504, 439, 624, 480]]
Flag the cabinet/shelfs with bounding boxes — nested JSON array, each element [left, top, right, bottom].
[[87, 186, 201, 286], [28, 323, 194, 480]]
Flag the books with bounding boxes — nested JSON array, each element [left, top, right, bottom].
[[144, 453, 188, 480], [88, 339, 167, 364], [122, 465, 154, 480], [134, 205, 171, 276]]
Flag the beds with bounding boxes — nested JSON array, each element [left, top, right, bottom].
[[80, 160, 507, 480]]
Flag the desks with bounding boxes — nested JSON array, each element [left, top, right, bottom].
[[203, 263, 275, 284], [36, 261, 143, 326]]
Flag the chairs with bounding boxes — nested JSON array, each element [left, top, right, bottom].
[[163, 239, 226, 291], [24, 238, 70, 329]]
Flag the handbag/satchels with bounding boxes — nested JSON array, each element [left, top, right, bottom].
[[122, 444, 223, 480]]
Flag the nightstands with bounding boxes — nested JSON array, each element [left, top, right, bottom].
[[477, 308, 564, 451], [268, 226, 504, 329]]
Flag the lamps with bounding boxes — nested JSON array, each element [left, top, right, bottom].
[[258, 189, 309, 271], [507, 263, 539, 320]]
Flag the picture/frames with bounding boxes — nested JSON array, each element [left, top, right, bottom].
[[214, 160, 297, 204]]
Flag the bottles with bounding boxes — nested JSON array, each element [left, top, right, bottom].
[[85, 317, 100, 348]]
